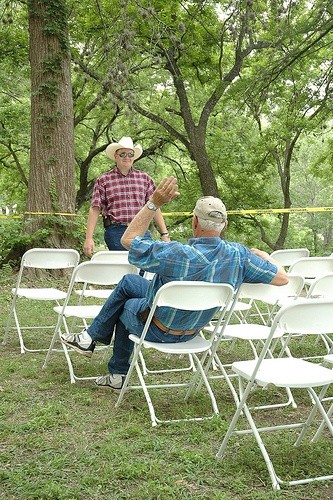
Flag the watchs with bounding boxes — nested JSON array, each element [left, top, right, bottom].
[[146, 201, 159, 212]]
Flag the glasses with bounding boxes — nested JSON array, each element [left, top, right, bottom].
[[117, 152, 134, 157]]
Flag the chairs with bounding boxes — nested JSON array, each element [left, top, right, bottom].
[[41, 248, 333, 490], [1, 247, 81, 356]]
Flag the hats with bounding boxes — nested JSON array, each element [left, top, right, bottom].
[[106, 136, 142, 160], [193, 196, 227, 223]]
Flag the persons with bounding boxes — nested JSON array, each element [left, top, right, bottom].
[[83, 136, 172, 258], [59, 175, 289, 395]]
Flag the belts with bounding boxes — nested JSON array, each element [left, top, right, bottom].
[[152, 317, 196, 335]]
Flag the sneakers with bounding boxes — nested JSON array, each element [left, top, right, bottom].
[[94, 373, 128, 391], [60, 328, 97, 358]]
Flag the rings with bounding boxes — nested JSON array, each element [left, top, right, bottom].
[[162, 187, 165, 190]]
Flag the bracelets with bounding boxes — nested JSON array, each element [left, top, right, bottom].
[[161, 231, 169, 236]]
